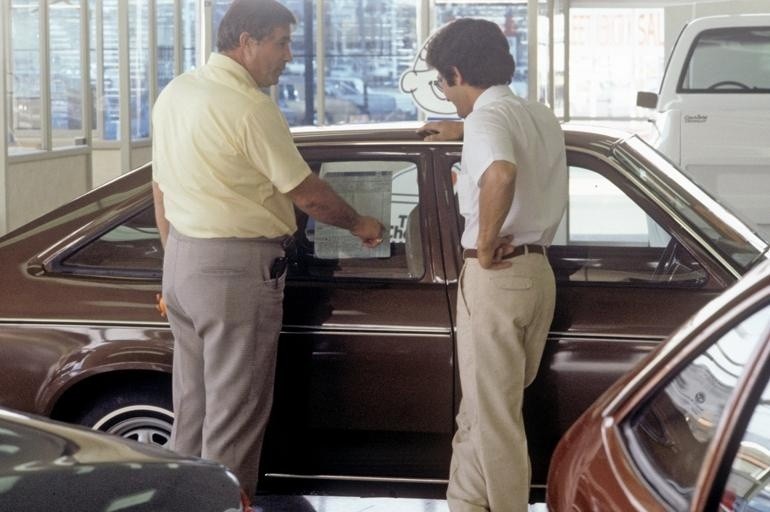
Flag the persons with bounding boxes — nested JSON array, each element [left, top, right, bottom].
[[148, 0, 393, 494], [414, 15, 573, 511]]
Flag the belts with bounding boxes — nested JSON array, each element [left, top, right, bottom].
[[463, 244, 552, 259]]
